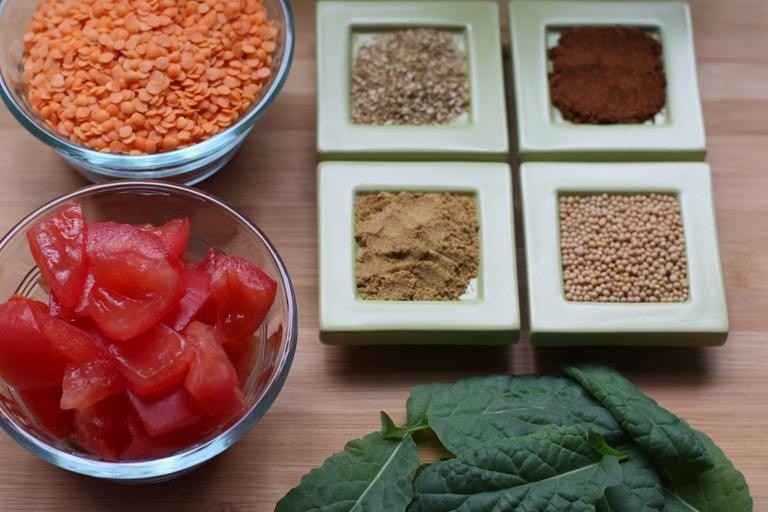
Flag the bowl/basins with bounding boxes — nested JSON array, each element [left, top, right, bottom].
[[0, 177, 301, 485], [1, 0, 297, 193]]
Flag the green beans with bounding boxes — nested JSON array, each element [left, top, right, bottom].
[[559, 192, 689, 303]]
